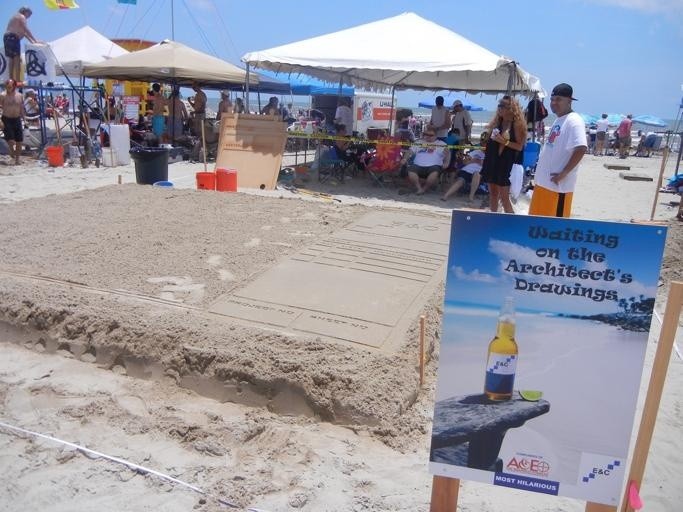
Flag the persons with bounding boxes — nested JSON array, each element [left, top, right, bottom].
[[0, 77, 668, 218], [3, 5, 37, 81]]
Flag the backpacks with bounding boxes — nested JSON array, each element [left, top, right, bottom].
[[526, 97, 548, 124]]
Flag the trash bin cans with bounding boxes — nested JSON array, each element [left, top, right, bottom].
[[130, 148, 169, 184]]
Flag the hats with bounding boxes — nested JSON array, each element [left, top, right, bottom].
[[549, 82, 579, 102], [218, 88, 230, 97], [451, 99, 462, 109]]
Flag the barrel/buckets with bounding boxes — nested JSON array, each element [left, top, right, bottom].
[[153, 179, 176, 189], [100, 146, 113, 167], [111, 122, 130, 166], [196, 171, 216, 190], [521, 139, 540, 170], [292, 122, 302, 134], [216, 167, 238, 191], [45, 145, 65, 167], [314, 144, 329, 160], [68, 145, 85, 165]]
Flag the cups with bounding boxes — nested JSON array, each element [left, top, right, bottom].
[[490, 128, 500, 140]]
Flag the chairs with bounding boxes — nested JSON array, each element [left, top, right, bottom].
[[316, 139, 541, 201]]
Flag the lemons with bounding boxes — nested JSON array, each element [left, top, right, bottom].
[[518, 390, 543, 401]]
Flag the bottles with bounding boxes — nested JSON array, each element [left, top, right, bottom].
[[483, 297, 516, 401]]
